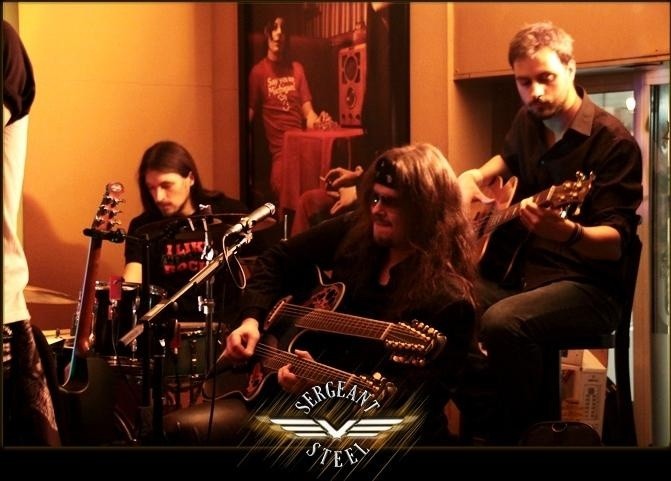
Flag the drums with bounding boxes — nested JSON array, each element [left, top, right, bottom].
[[72, 279, 177, 367], [167, 322, 229, 392]]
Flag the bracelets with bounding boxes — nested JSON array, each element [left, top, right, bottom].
[[562, 222, 584, 248]]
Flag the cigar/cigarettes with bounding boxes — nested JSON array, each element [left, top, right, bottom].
[[319, 176, 331, 184]]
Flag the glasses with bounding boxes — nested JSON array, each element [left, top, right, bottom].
[[366, 189, 406, 211]]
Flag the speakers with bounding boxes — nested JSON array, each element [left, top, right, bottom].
[[338, 43, 367, 126]]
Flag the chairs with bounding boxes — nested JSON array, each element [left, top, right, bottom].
[[511, 216, 642, 447]]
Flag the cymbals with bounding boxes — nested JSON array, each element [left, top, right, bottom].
[[22, 285, 81, 306]]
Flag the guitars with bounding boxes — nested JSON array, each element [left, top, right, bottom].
[[460, 170, 597, 265], [55, 184, 138, 446], [200, 265, 448, 417]]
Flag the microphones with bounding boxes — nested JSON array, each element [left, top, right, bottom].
[[224, 203, 276, 237]]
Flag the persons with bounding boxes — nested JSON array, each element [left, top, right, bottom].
[[122, 142, 263, 357], [161, 142, 477, 447], [247, 10, 332, 202], [288, 162, 367, 236], [458, 21, 643, 445], [3, 21, 63, 446]]
